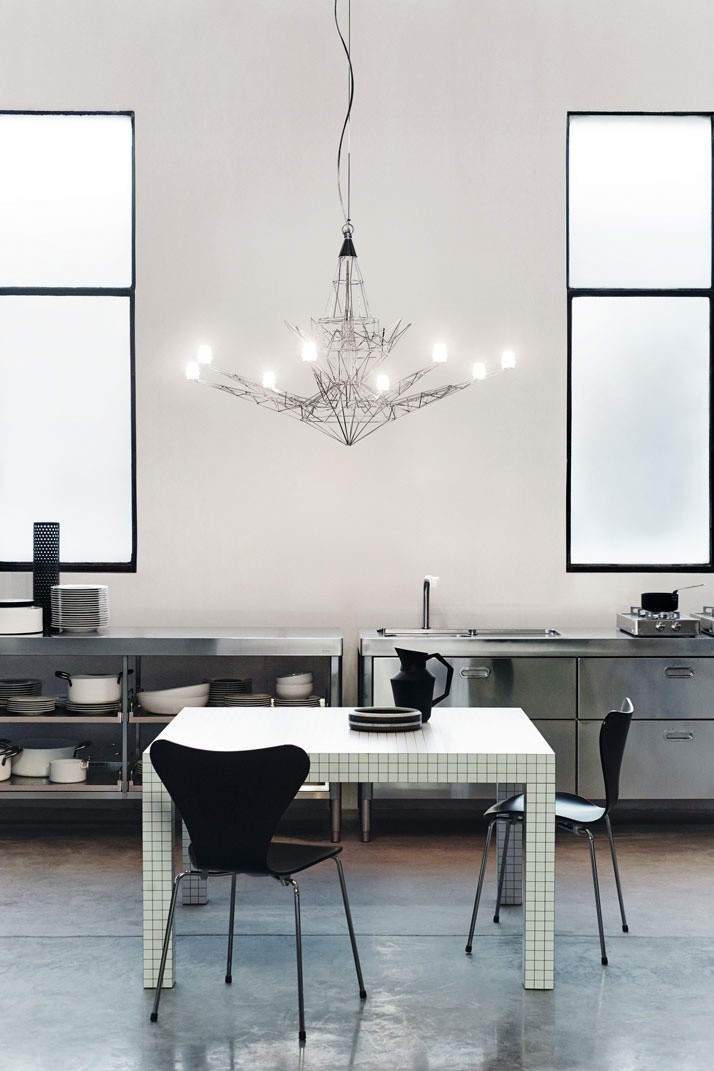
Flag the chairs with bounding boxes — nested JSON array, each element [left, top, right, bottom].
[[460, 698, 634, 966], [146, 739, 368, 1047]]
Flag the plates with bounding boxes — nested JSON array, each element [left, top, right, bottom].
[[139, 679, 320, 716], [348, 706, 421, 730], [1, 678, 54, 714], [48, 584, 111, 633], [57, 694, 122, 715]]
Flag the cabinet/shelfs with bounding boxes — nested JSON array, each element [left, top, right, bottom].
[[361, 654, 577, 806], [576, 659, 714, 802], [1, 625, 346, 844]]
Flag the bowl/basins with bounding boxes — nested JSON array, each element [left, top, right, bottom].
[[277, 671, 313, 700]]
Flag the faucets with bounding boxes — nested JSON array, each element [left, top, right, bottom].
[[421, 575, 432, 630]]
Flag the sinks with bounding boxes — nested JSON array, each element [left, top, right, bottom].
[[378, 626, 473, 638], [471, 627, 561, 639]]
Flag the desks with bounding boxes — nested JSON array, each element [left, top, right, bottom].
[[141, 705, 557, 993]]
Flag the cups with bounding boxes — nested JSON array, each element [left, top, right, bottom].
[[390, 647, 454, 722]]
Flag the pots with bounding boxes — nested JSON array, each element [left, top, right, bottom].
[[53, 669, 136, 705], [641, 583, 705, 610], [0, 739, 120, 783]]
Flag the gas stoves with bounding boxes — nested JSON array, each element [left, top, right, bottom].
[[617, 604, 714, 637]]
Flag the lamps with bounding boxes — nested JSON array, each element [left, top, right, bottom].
[[182, 0, 521, 446]]
